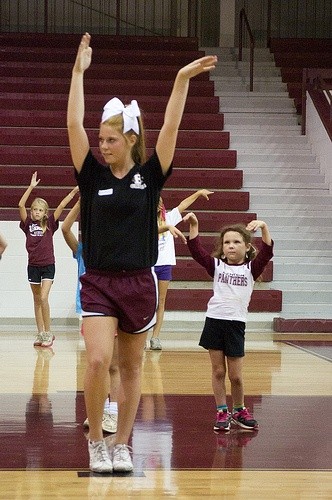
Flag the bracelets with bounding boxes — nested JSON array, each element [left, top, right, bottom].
[[68, 194, 73, 199]]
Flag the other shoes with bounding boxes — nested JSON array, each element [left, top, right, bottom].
[[34, 331, 56, 347], [150, 336, 162, 351]]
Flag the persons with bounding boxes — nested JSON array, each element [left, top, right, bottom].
[[18, 170, 80, 348], [59, 194, 188, 434], [182, 211, 274, 434], [144, 188, 215, 351], [64, 30, 218, 477]]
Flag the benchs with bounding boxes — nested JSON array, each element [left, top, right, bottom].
[[0, 32, 332, 334]]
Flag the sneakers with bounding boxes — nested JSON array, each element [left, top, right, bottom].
[[212, 409, 232, 431], [87, 437, 113, 474], [82, 417, 90, 427], [100, 407, 120, 434], [106, 441, 135, 472], [228, 407, 259, 431]]
